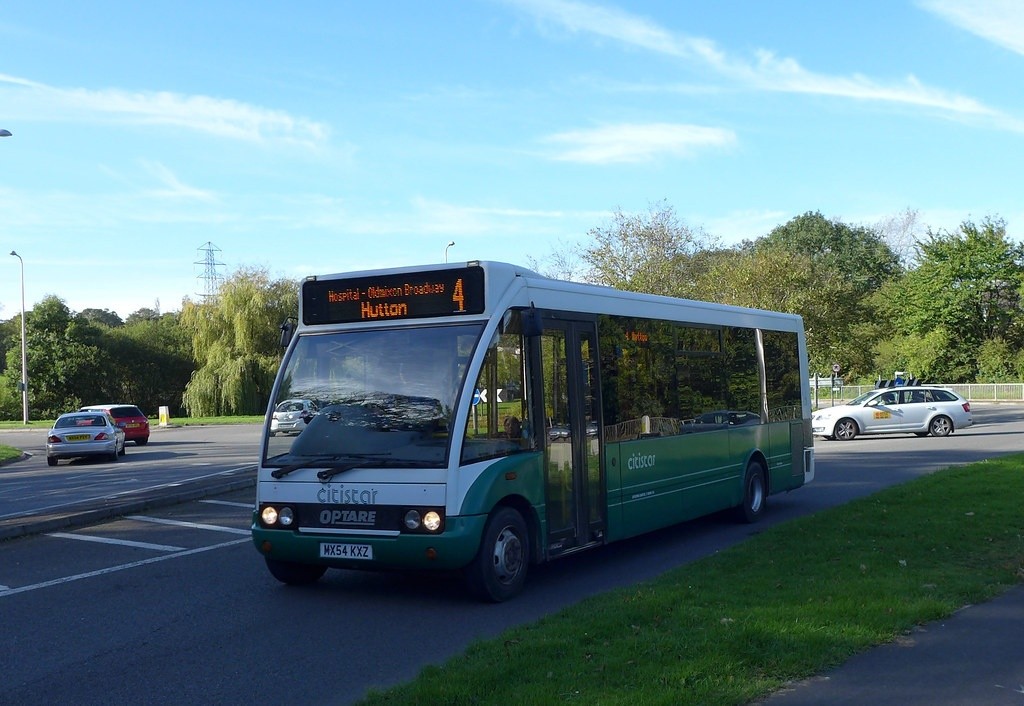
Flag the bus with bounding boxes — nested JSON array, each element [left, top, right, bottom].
[[250, 261, 816, 605]]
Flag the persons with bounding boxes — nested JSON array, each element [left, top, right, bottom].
[[500, 417, 522, 439], [398, 356, 420, 387], [881, 392, 893, 403], [910, 390, 921, 403]]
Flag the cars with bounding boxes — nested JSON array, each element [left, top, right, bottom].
[[76, 404, 150, 446], [680, 410, 762, 435], [270, 399, 320, 437], [811, 377, 972, 442], [46, 413, 126, 467]]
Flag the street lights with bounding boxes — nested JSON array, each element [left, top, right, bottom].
[[10, 251, 29, 425], [445, 242, 455, 264]]
